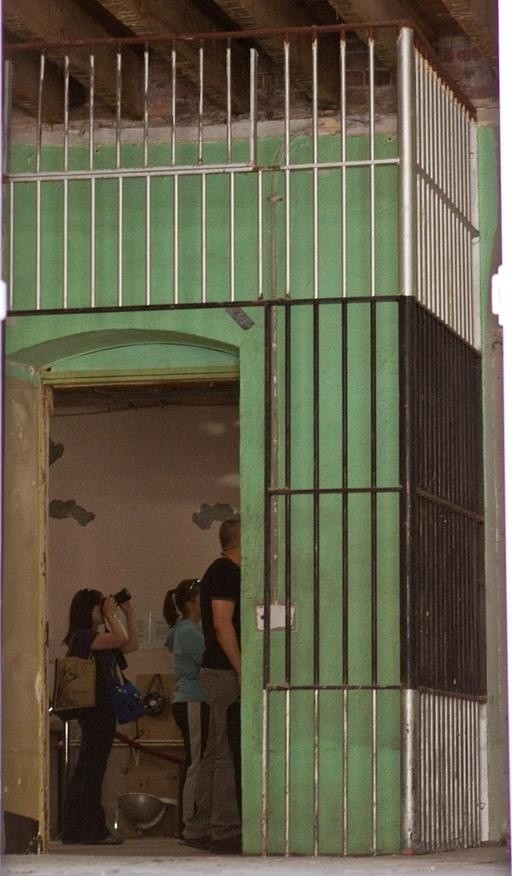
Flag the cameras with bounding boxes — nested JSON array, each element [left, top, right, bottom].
[[101, 588, 131, 606]]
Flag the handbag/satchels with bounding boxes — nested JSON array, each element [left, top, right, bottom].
[[53, 656, 96, 711], [114, 683, 146, 725]]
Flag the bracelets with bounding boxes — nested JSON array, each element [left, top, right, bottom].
[[107, 614, 117, 620]]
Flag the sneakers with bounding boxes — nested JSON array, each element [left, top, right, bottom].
[[185, 835, 240, 854], [62, 831, 125, 844]]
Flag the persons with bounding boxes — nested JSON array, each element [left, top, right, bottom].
[[164, 579, 207, 839], [182, 513, 241, 856], [53, 587, 143, 846]]
